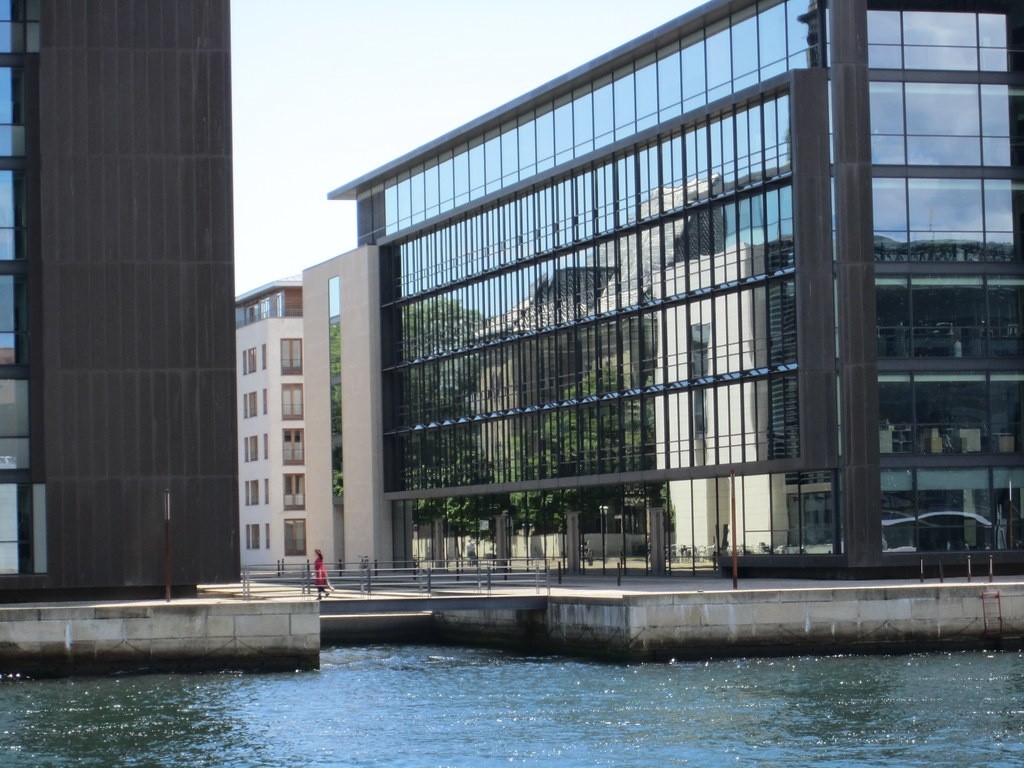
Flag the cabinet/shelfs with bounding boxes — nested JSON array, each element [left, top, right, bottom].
[[880, 421, 1014, 453]]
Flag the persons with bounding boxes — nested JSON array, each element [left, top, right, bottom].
[[313, 548, 329, 600], [466, 539, 475, 566]]
